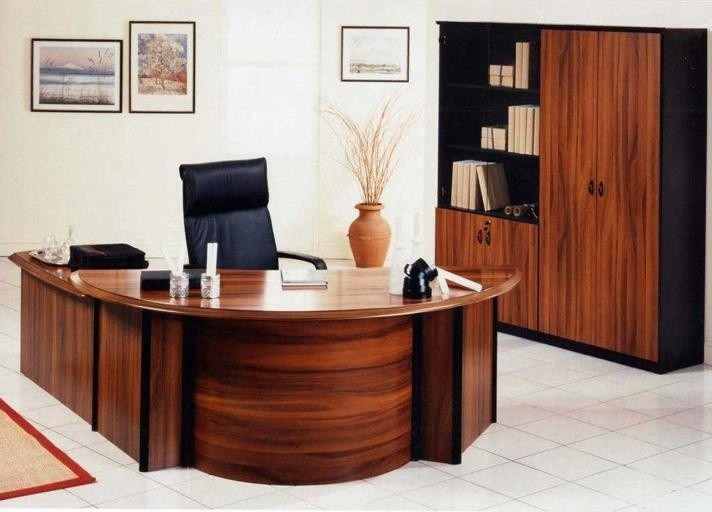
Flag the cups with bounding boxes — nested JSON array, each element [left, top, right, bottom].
[[45, 233, 70, 264], [199, 274, 220, 299], [169, 273, 189, 298]]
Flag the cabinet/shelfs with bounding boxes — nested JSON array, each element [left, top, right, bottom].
[[430, 19, 705, 373]]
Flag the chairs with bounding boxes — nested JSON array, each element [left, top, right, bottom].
[[178, 156, 328, 273]]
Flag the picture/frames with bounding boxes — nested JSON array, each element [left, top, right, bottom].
[[339, 25, 410, 84], [29, 22, 197, 114]]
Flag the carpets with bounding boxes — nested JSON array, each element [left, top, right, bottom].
[[0, 396, 95, 499]]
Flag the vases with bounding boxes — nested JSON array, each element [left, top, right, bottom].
[[348, 202, 392, 270]]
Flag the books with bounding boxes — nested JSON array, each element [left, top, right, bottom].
[[281, 267, 329, 290], [479, 101, 539, 156], [489, 40, 539, 89], [450, 159, 511, 212]]
